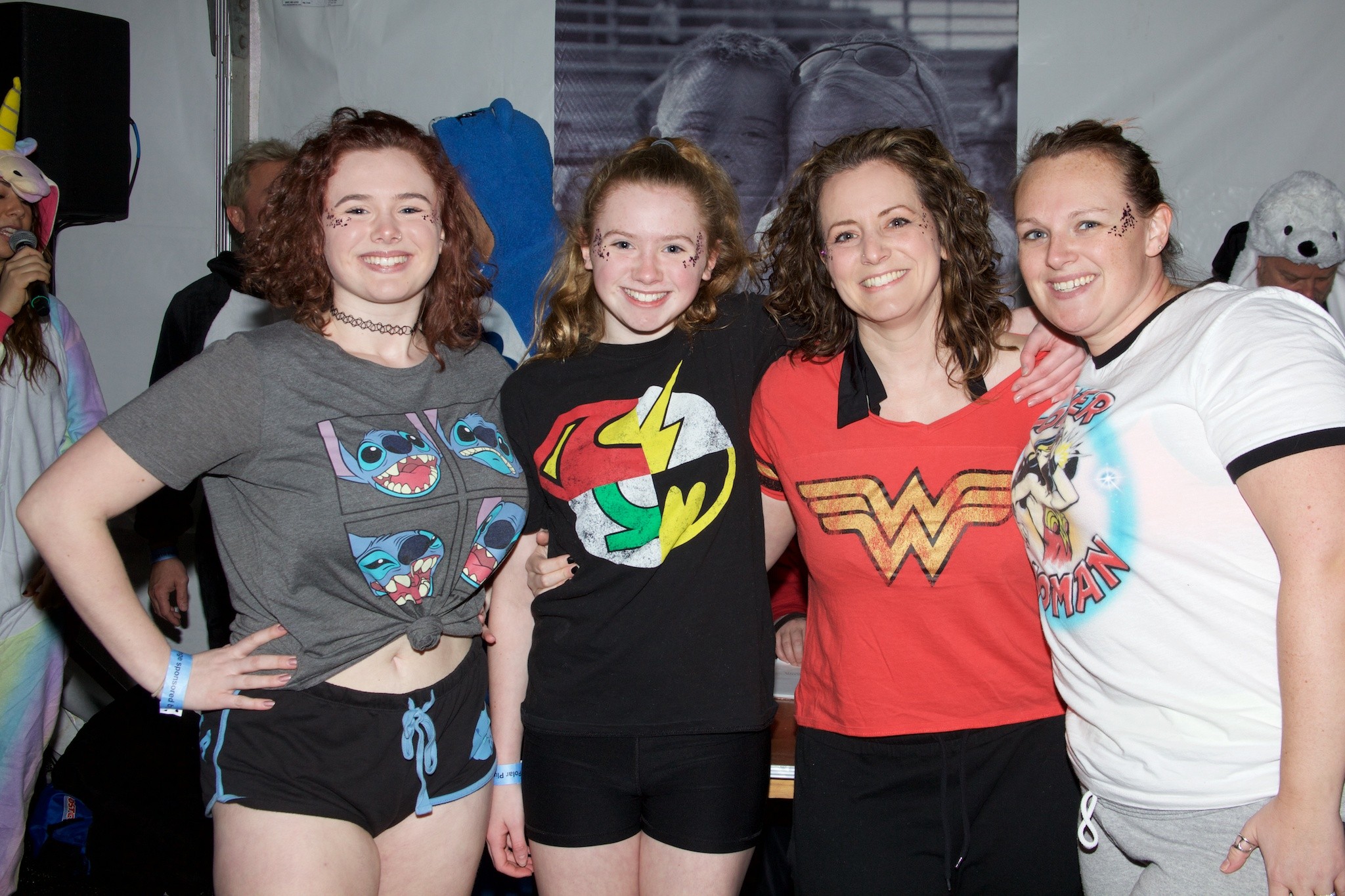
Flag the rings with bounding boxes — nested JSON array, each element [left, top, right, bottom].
[[1314, 890, 1336, 896], [1232, 833, 1257, 852]]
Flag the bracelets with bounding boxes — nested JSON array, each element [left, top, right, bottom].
[[154, 553, 178, 562], [492, 759, 523, 785], [149, 648, 193, 717]]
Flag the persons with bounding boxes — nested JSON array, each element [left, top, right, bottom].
[[1010, 117, 1345, 896], [485, 139, 1087, 894], [147, 136, 303, 652], [0, 76, 108, 895], [629, 22, 1018, 312], [1228, 170, 1345, 336], [526, 122, 1085, 895], [16, 108, 529, 895]]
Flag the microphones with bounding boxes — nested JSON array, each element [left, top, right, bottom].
[[8, 230, 50, 316]]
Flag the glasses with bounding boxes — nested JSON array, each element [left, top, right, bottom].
[[789, 40, 922, 93]]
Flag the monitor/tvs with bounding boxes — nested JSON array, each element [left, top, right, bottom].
[[0, 2, 132, 228]]
